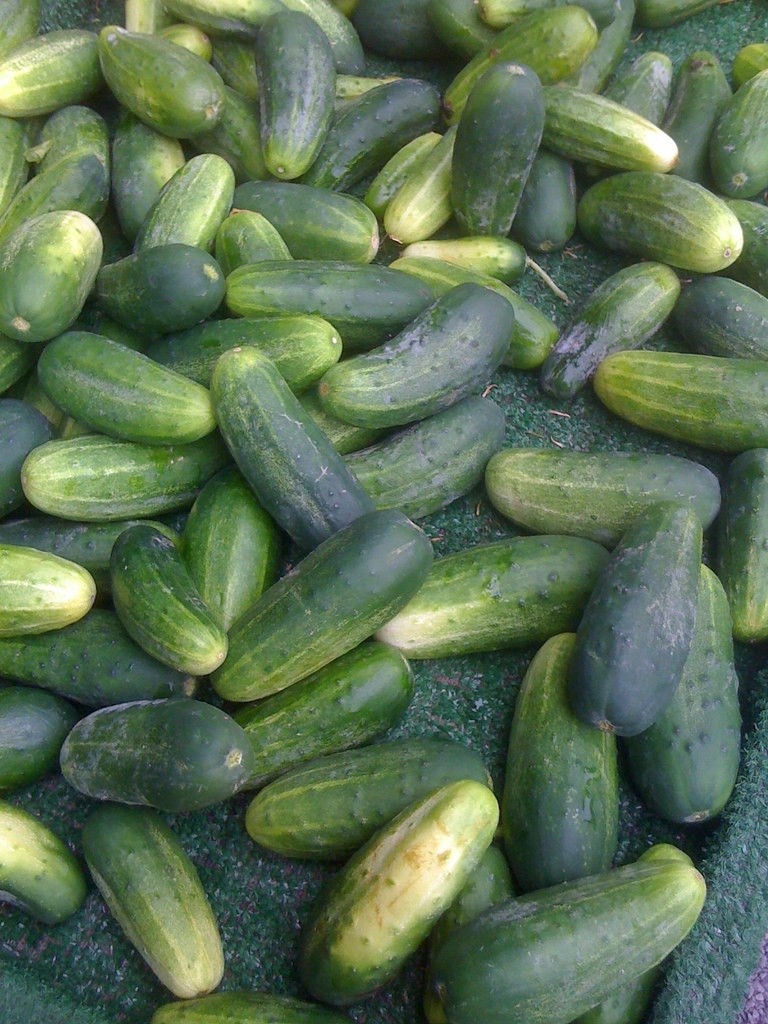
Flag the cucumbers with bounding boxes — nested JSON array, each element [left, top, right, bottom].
[[0, 0, 768, 1024]]
